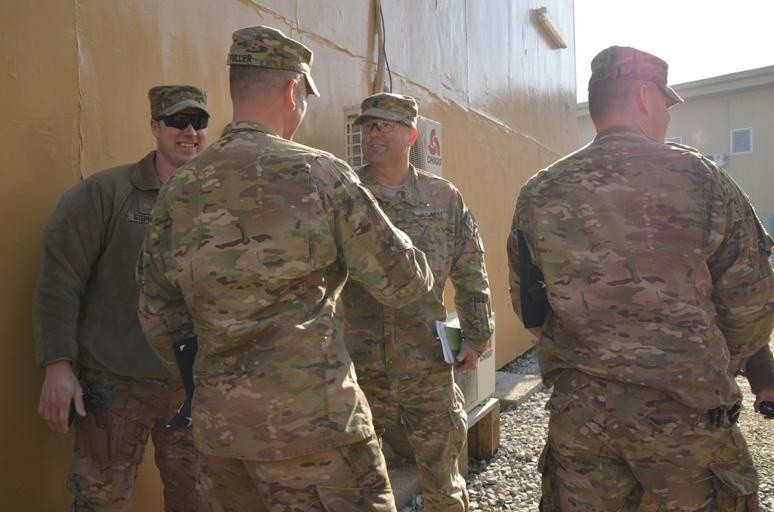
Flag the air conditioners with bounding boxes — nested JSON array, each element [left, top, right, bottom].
[[445, 308, 497, 415], [343, 103, 444, 179], [706, 152, 727, 167]]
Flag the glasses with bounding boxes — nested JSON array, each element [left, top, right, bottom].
[[359, 119, 408, 132], [163, 113, 208, 130]]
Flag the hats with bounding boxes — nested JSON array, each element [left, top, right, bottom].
[[353, 92, 418, 129], [587, 46, 684, 108], [225, 25, 320, 99], [148, 85, 210, 119]]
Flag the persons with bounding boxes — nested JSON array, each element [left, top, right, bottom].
[[336, 92, 496, 512], [136, 25, 436, 512], [36, 83, 214, 511], [506, 44, 774, 512]]
